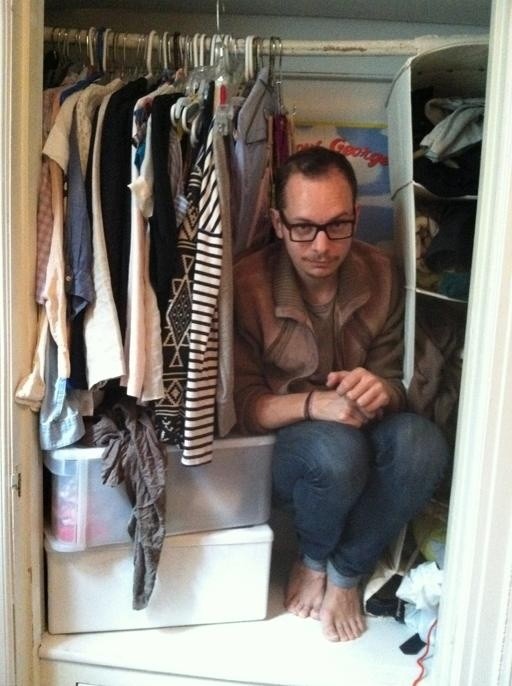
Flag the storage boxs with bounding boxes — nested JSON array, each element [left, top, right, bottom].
[[40, 524, 276, 634], [40, 432, 279, 554]]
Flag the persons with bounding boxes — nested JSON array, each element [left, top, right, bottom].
[[232, 146, 449, 642]]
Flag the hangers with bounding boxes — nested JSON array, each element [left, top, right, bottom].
[[44, 26, 277, 147]]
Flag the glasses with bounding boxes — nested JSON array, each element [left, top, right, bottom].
[[278, 206, 356, 243]]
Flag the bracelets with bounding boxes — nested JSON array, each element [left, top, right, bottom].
[[303, 390, 315, 421]]
